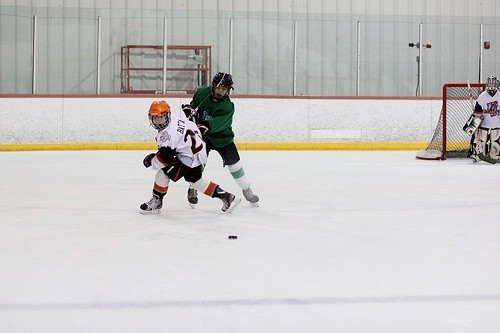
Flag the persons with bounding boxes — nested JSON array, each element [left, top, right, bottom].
[[138, 100, 241, 215], [463, 75, 500, 164], [182, 72, 260, 209]]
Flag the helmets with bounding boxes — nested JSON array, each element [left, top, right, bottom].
[[213, 72, 234, 87], [486, 75, 498, 92], [148, 101, 171, 131]]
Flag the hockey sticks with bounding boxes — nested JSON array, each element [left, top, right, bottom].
[[187, 92, 213, 121], [467, 78, 497, 164]]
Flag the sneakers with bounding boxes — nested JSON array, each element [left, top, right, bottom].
[[242, 187, 259, 207], [211, 185, 241, 213], [139, 190, 166, 214], [187, 188, 198, 209]]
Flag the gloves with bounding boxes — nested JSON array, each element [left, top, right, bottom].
[[143, 153, 156, 168]]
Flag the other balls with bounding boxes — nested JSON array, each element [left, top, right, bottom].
[[229, 236, 238, 239]]
